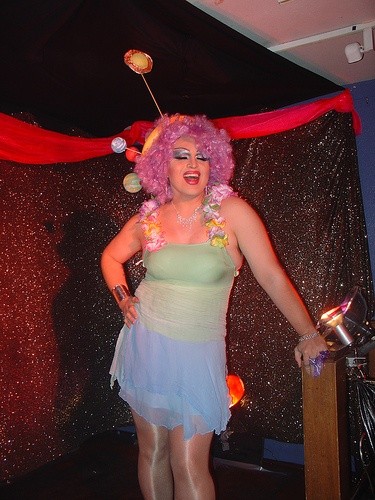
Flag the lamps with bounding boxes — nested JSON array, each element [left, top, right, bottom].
[[320, 306, 354, 346], [344, 28, 373, 64]]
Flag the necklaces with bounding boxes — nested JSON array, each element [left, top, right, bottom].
[[171, 199, 203, 232]]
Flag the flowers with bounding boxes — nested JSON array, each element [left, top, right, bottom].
[[136, 183, 238, 252]]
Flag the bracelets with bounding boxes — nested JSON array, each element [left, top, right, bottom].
[[298, 331, 319, 342], [111, 284, 131, 310]]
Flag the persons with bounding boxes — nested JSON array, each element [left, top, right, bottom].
[[100, 112, 327, 500]]
